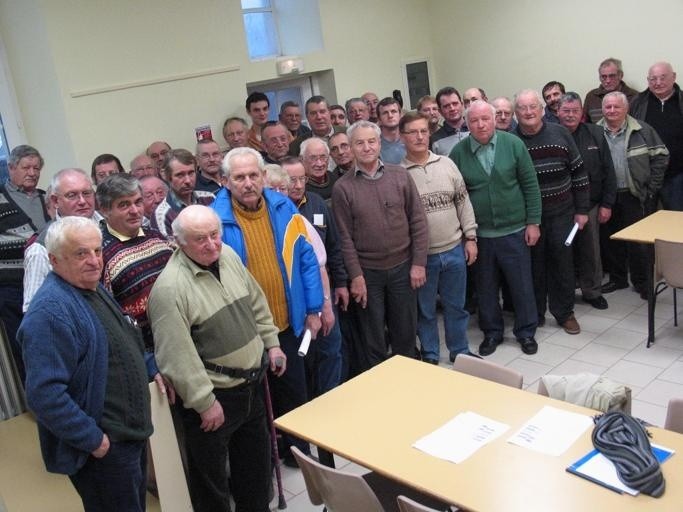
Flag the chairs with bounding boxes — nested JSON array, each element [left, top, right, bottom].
[[650, 239, 683, 342], [289, 446, 453, 512], [452, 354, 523, 389], [537, 375, 631, 416], [396, 495, 441, 512], [664, 399, 683, 434]]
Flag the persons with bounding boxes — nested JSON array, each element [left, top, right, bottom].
[[13, 214, 176, 510], [1, 57, 679, 386], [143, 202, 288, 511], [205, 145, 327, 470]]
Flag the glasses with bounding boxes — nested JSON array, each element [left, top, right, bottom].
[[53, 190, 93, 199], [289, 176, 310, 184]]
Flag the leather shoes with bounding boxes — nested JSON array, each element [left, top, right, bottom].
[[600, 280, 629, 293], [636, 285, 650, 300], [517, 335, 538, 354], [450, 352, 483, 363], [479, 335, 504, 356], [582, 293, 608, 310]]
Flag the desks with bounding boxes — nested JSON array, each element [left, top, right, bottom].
[[609, 210, 683, 348], [273, 354, 683, 512]]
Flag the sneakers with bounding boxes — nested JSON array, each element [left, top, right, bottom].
[[562, 316, 581, 334], [538, 315, 546, 326], [502, 304, 517, 317]]
